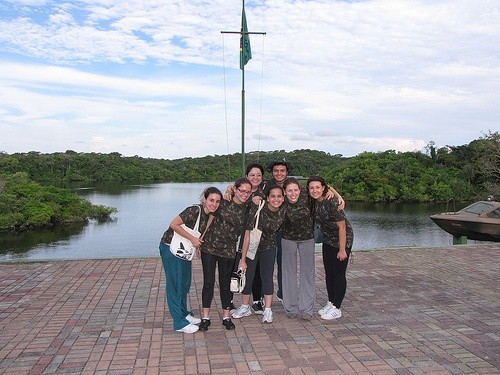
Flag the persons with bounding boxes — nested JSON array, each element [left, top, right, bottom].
[[252, 180, 334, 324], [308, 176, 354, 319], [226, 163, 345, 307], [159, 187, 222, 333], [195, 178, 256, 331], [223, 164, 265, 314]]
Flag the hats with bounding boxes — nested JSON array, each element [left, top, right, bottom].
[[266, 160, 289, 172]]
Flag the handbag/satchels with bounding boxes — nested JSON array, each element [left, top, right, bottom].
[[236, 198, 265, 260], [169, 205, 202, 262], [230, 270, 247, 293], [313, 222, 322, 243]]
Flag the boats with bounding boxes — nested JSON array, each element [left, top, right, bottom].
[[430, 194, 500, 243]]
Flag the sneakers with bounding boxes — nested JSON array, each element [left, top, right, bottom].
[[232, 306, 252, 318], [230, 302, 236, 310], [318, 301, 333, 315], [200, 319, 211, 331], [223, 319, 235, 330], [262, 310, 273, 324], [252, 302, 263, 314], [185, 314, 201, 324], [321, 304, 342, 320], [175, 323, 199, 334]]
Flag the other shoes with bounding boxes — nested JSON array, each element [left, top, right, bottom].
[[301, 313, 311, 321], [287, 313, 297, 319], [276, 293, 283, 301]]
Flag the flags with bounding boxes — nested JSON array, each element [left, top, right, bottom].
[[240, 10, 252, 69]]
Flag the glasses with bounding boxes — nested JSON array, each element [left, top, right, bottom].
[[237, 187, 251, 195]]
